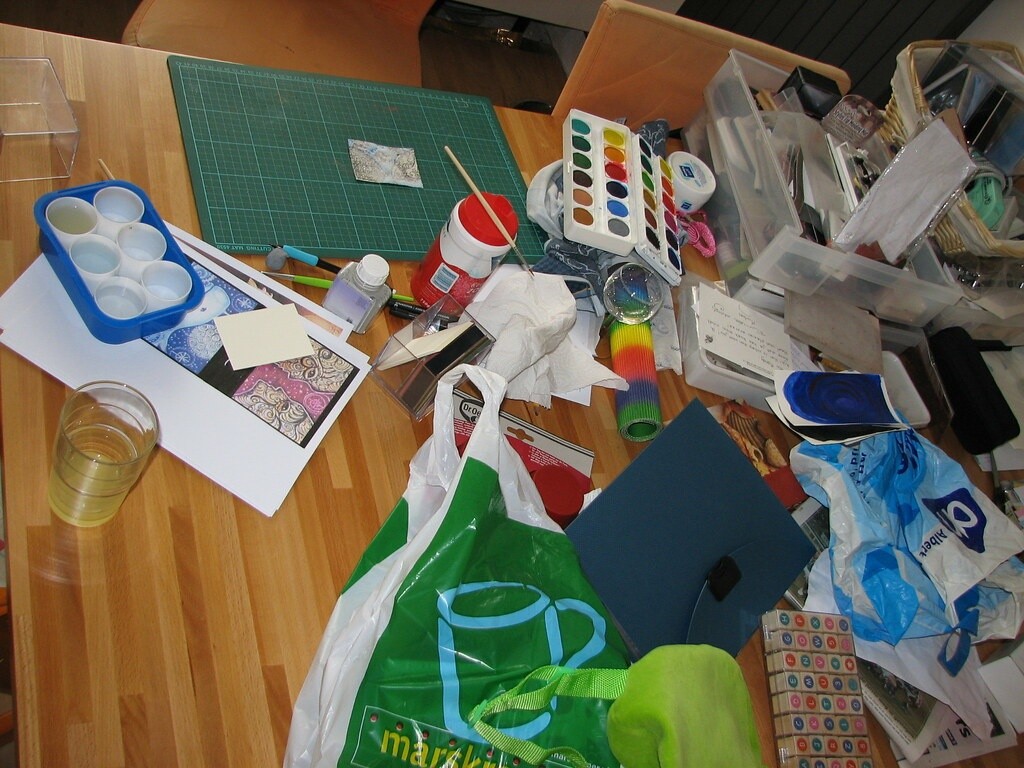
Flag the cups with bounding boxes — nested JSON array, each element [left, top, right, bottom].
[[68, 233, 122, 296], [95, 276, 148, 320], [46, 196, 99, 254], [117, 222, 167, 283], [93, 186, 145, 242], [48, 381, 160, 527], [141, 261, 192, 313]]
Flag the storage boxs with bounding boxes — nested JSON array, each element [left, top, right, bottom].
[[683, 47, 1024, 417]]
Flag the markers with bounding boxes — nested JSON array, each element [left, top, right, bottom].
[[389, 301, 460, 330]]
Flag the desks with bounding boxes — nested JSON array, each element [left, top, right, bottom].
[[0, 22, 1024, 768]]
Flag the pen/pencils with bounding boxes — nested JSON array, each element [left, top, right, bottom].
[[261, 270, 415, 303], [269, 242, 396, 295], [445, 145, 534, 277]]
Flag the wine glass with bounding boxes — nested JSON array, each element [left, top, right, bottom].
[[595, 263, 664, 359]]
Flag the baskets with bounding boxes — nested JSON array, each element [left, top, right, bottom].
[[876, 39, 1024, 257]]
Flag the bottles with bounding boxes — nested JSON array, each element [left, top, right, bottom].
[[322, 254, 392, 334], [410, 193, 519, 317]]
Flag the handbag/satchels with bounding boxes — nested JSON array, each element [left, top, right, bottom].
[[282, 363, 637, 768], [473, 642, 750, 768], [790, 410, 1024, 676]]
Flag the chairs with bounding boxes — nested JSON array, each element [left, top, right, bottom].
[[551, 0, 850, 140], [122, 0, 436, 88]]
